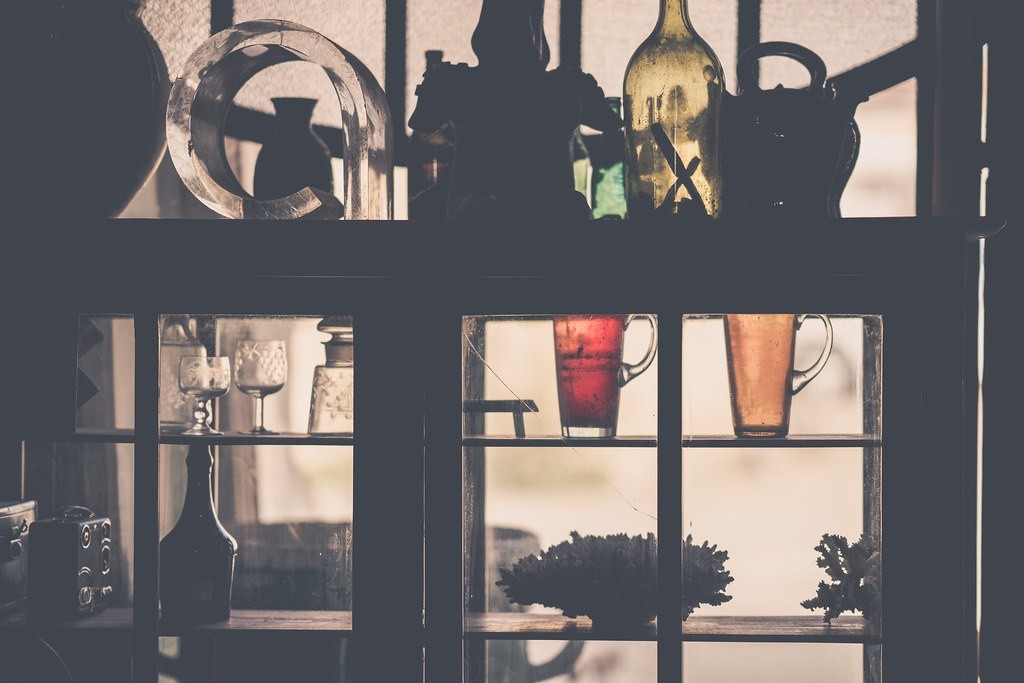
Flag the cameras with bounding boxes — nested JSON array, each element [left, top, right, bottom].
[[0, 496, 112, 620]]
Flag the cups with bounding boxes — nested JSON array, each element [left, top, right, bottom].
[[723, 314, 833, 438], [552, 313, 658, 438]]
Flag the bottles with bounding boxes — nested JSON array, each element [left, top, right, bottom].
[[252, 97, 336, 198], [572, 125, 589, 199], [407, 50, 455, 220], [591, 98, 628, 221], [159, 444, 238, 626], [160, 315, 207, 435], [621, 1, 726, 221], [308, 317, 354, 436], [699, 40, 861, 218]]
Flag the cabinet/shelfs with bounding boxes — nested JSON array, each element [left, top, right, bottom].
[[0, 272, 983, 683]]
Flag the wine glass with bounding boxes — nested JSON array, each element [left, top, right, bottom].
[[180, 355, 231, 436], [233, 339, 288, 436]]
